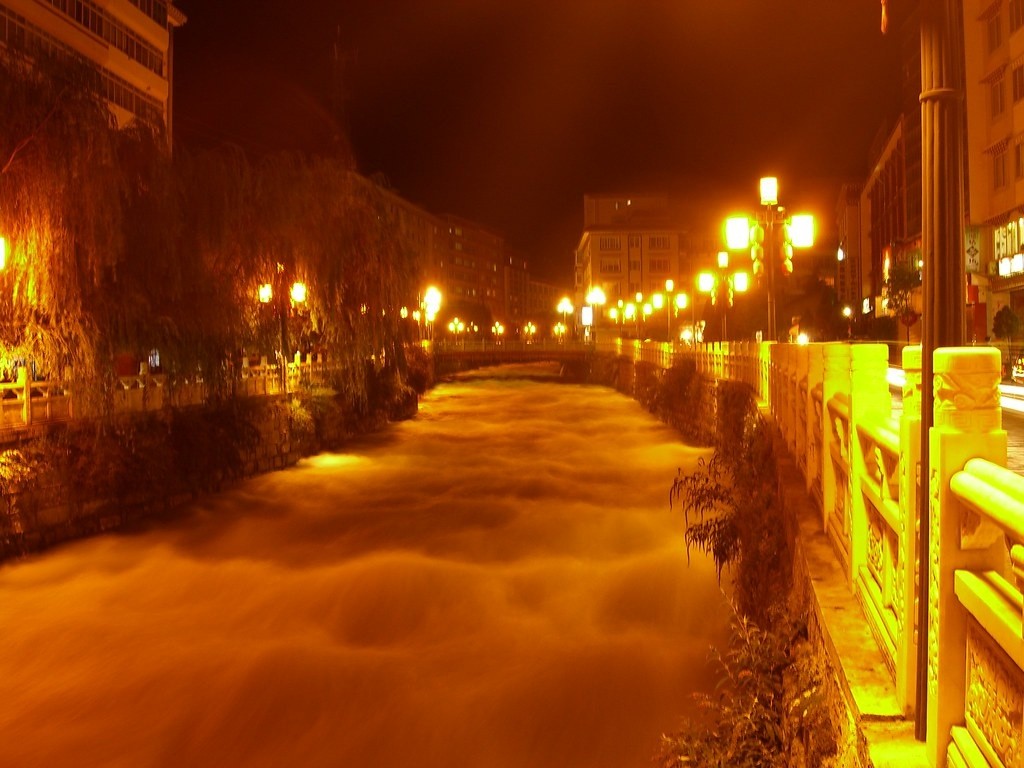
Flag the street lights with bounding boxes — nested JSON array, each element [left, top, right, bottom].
[[256, 259, 307, 390], [344, 289, 537, 346], [555, 270, 754, 345], [719, 176, 817, 342]]
